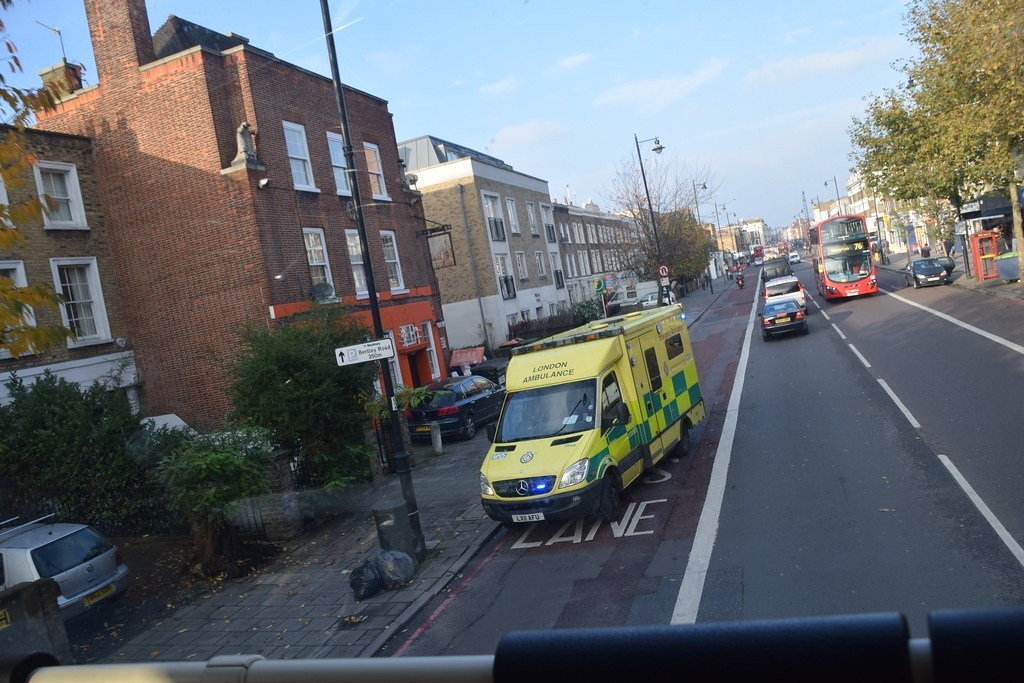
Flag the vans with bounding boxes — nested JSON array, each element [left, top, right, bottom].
[[763, 275, 809, 314]]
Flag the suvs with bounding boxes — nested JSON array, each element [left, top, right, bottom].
[[789, 250, 802, 265], [408, 373, 506, 443]]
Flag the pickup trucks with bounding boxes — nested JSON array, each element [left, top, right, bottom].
[[0, 513, 133, 628]]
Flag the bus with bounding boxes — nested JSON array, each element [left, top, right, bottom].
[[809, 214, 878, 300]]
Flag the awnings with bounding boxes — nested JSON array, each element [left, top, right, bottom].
[[450, 347, 484, 366]]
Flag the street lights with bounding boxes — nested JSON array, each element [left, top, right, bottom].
[[692, 182, 714, 297], [715, 204, 726, 286], [633, 132, 672, 305], [824, 176, 844, 216]]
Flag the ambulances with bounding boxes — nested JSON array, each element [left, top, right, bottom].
[[479, 300, 709, 526]]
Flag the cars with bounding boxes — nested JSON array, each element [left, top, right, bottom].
[[754, 257, 764, 266], [758, 298, 809, 342], [902, 256, 957, 290]]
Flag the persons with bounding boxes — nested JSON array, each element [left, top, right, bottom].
[[920, 244, 931, 259], [944, 238, 951, 257], [872, 239, 890, 255], [993, 224, 1001, 238], [736, 272, 744, 285], [803, 243, 812, 256]]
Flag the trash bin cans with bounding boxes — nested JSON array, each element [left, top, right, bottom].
[[993, 251, 1020, 285], [371, 500, 417, 568]]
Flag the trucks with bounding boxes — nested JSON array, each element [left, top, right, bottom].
[[754, 246, 764, 258], [761, 257, 794, 283]]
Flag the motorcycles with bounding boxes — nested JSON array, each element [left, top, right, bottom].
[[736, 278, 746, 288]]
